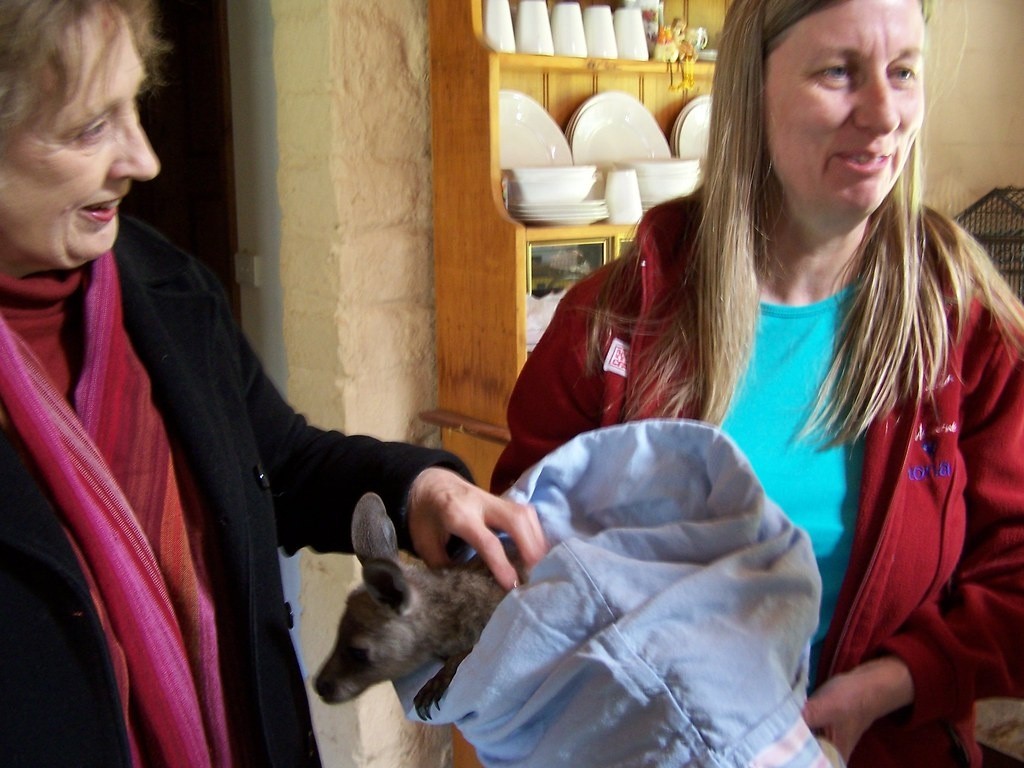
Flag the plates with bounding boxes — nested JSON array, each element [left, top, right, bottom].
[[671, 94, 712, 159], [509, 199, 610, 227], [566, 90, 670, 179], [697, 50, 719, 62], [641, 201, 663, 215], [499, 88, 572, 166]]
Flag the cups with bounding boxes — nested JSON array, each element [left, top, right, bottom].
[[613, 8, 649, 60], [683, 27, 708, 50], [551, 1, 588, 58], [482, 0, 516, 53], [516, 0, 554, 56], [586, 170, 606, 198], [582, 4, 618, 60], [605, 169, 643, 226]]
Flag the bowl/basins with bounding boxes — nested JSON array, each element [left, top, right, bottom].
[[507, 180, 596, 201], [501, 165, 596, 180], [614, 156, 700, 200]]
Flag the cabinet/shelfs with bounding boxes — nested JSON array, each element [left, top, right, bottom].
[[427, 0, 731, 768]]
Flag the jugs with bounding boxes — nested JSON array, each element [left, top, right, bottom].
[[622, 0, 664, 60]]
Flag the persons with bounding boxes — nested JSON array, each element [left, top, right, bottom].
[[490, 0, 1024, 768], [0, 0, 546, 768]]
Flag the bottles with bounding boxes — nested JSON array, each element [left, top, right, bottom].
[[654, 25, 679, 63]]
